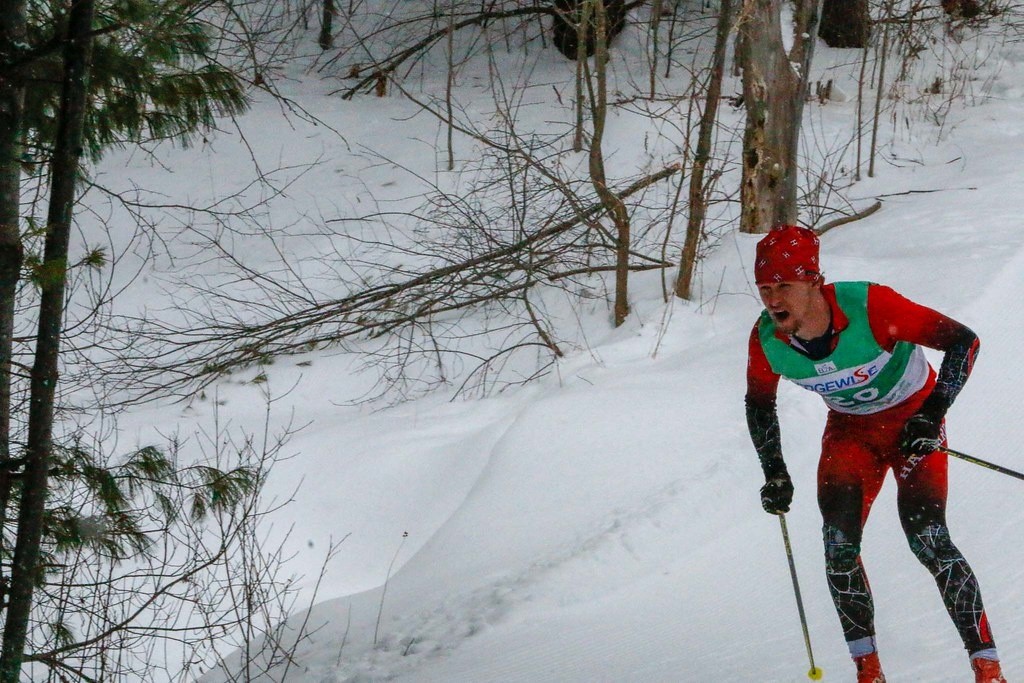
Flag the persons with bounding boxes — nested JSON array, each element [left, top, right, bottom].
[[745, 226, 1008, 683]]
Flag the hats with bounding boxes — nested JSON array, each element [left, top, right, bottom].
[[754, 224, 825, 284]]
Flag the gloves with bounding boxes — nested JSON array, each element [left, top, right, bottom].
[[896, 406, 943, 460], [760, 462, 794, 514]]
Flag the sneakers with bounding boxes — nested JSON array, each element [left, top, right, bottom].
[[972, 658, 1007, 683], [853, 652, 886, 683]]
